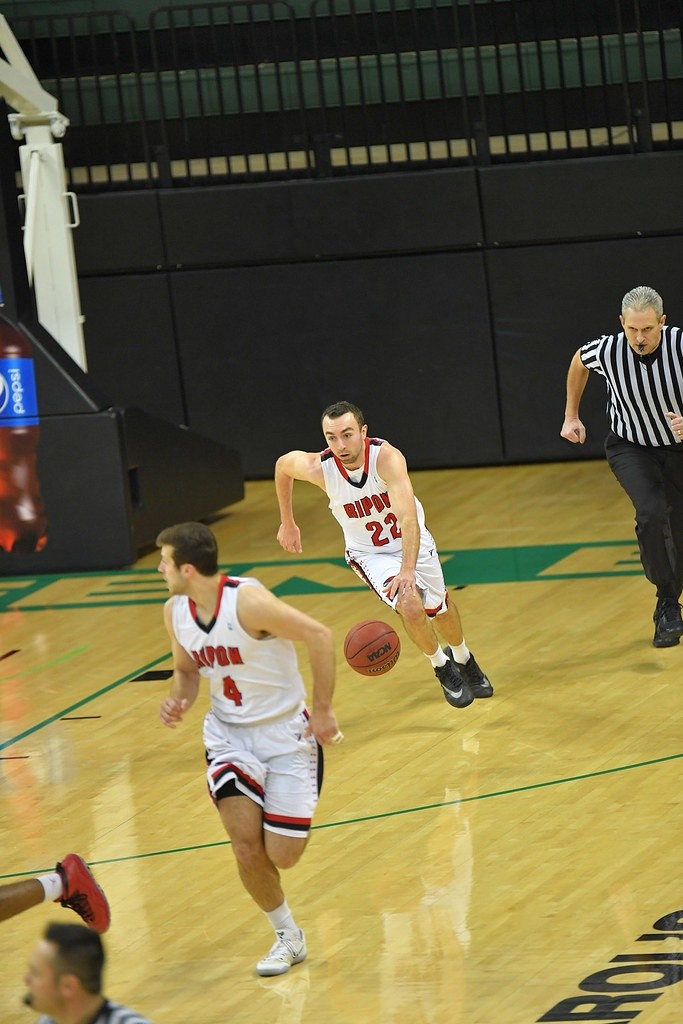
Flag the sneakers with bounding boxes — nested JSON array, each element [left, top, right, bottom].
[[653, 596, 683, 647], [433, 657, 474, 709], [256, 929, 306, 976], [446, 646, 493, 698], [53, 855, 110, 934]]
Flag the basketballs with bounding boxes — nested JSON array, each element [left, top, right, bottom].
[[341, 619, 403, 678]]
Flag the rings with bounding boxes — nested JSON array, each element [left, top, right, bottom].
[[678, 430, 681, 434]]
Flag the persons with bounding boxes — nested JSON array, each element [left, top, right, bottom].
[[0, 853, 112, 935], [274, 401, 494, 709], [559, 286, 683, 647], [23, 920, 149, 1024], [155, 523, 345, 976]]
